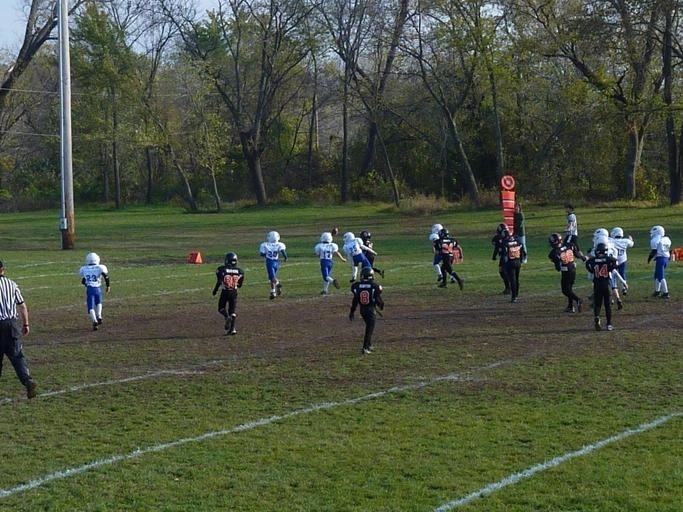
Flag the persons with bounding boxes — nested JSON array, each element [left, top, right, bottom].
[[349, 267, 384, 354], [434, 228, 464, 289], [0, 260, 37, 399], [430, 223, 456, 283], [259, 231, 288, 299], [360, 231, 384, 279], [648, 225, 672, 298], [342, 232, 377, 282], [212, 253, 244, 335], [80, 252, 110, 331], [314, 232, 346, 295], [492, 204, 634, 332]]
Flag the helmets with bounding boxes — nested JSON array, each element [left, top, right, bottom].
[[611, 226, 624, 238], [320, 231, 332, 242], [549, 233, 562, 245], [86, 252, 100, 265], [344, 231, 371, 242], [431, 223, 450, 238], [497, 223, 513, 238], [649, 225, 665, 236], [593, 228, 609, 257], [360, 267, 374, 281], [225, 252, 237, 266], [266, 231, 280, 242]]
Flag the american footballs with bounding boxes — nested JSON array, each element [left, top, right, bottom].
[[332, 228, 338, 236]]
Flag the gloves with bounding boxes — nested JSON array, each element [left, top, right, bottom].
[[349, 311, 354, 321]]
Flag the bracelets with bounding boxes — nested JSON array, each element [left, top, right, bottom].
[[23, 324, 29, 327]]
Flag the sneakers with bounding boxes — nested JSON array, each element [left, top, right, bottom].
[[437, 275, 465, 290], [333, 279, 340, 289], [652, 291, 669, 299], [276, 284, 282, 296], [27, 382, 37, 399], [225, 317, 237, 335], [564, 298, 583, 313], [595, 317, 601, 331], [502, 288, 518, 303], [93, 320, 98, 330], [607, 325, 614, 330], [350, 279, 355, 282], [320, 291, 327, 294], [615, 280, 628, 311], [97, 315, 102, 324], [382, 270, 384, 278], [269, 292, 276, 299]]
